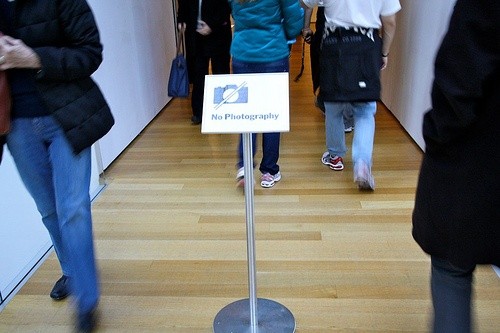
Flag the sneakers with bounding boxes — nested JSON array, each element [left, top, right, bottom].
[[235, 167, 245, 188], [353, 172, 374, 191], [261, 172, 281, 188], [322, 152, 344, 171]]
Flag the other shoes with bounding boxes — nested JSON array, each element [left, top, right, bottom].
[[344, 123, 354, 132], [314, 96, 326, 116]]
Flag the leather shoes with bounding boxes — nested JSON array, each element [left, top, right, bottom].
[[50, 275, 73, 300], [78, 313, 93, 333]]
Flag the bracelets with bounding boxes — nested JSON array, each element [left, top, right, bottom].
[[381, 52, 391, 57]]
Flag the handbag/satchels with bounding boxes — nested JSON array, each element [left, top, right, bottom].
[[168, 26, 189, 98]]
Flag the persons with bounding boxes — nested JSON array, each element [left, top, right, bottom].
[[228, 0, 305, 189], [0, 0, 116, 332], [177, 0, 232, 125], [411, 0, 500, 333], [300, 0, 402, 192]]
